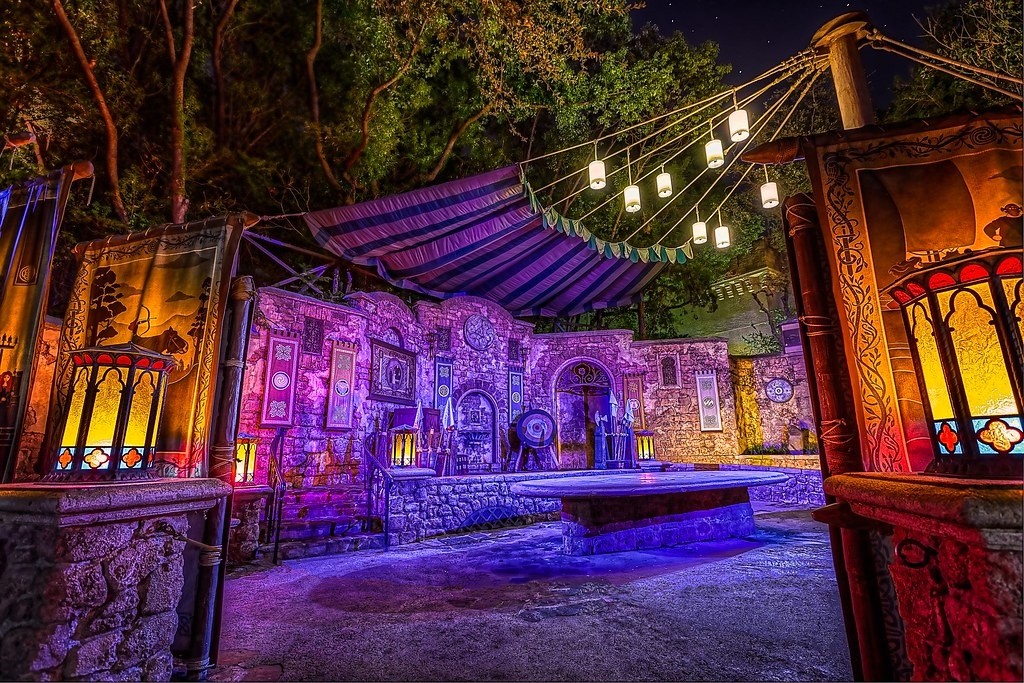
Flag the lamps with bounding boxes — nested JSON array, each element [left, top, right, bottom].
[[656, 164, 672, 198], [589, 140, 607, 190], [692, 204, 708, 244], [880, 244, 1024, 481], [388, 424, 419, 468], [35, 341, 174, 484], [715, 208, 731, 249], [729, 88, 749, 143], [705, 119, 725, 169], [624, 146, 641, 213], [760, 163, 780, 209], [635, 429, 655, 461], [234, 432, 263, 486]]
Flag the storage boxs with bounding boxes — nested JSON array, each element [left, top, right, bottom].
[[394, 408, 441, 433]]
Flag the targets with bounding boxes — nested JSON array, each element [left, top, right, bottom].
[[515, 408, 557, 448]]
